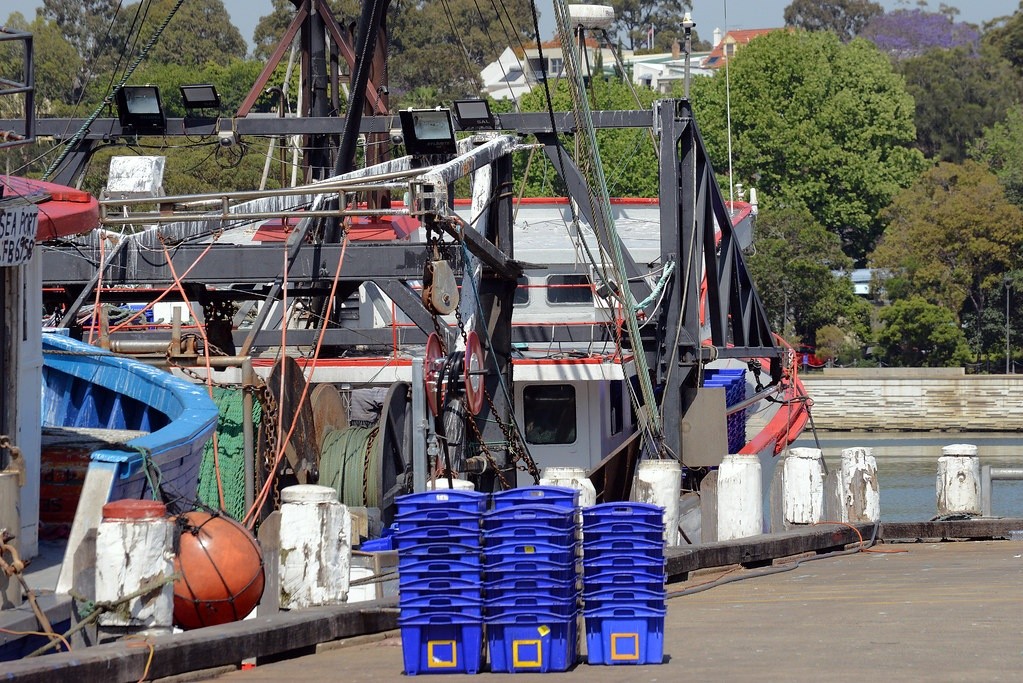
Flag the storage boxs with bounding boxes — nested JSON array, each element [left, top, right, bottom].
[[358, 485, 667, 678], [698, 368, 747, 458]]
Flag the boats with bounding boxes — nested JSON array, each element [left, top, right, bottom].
[[0, 174, 220, 661], [1, 1, 812, 546]]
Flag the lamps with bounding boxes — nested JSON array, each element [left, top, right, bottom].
[[399, 108, 458, 155], [112, 85, 168, 135], [453, 99, 495, 131], [180, 83, 223, 118]]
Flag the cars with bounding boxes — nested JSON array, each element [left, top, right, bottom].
[[790, 344, 836, 371]]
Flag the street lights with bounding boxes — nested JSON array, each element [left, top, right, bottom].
[[1003, 277, 1015, 374]]
